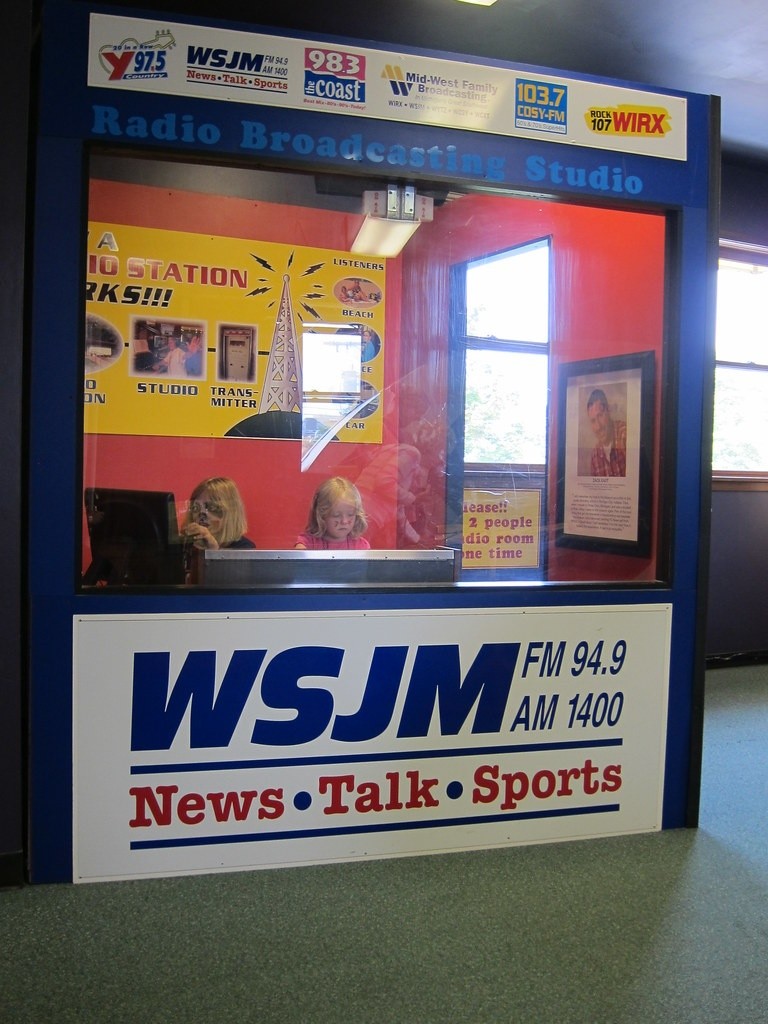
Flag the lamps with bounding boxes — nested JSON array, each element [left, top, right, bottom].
[[348, 193, 436, 257]]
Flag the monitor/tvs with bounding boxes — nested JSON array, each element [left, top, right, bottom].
[[85, 487, 185, 586]]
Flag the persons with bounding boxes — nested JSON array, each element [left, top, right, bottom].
[[180, 476, 256, 550], [587, 389, 626, 477], [152, 336, 201, 376], [294, 476, 370, 550], [361, 330, 375, 362], [339, 279, 369, 303]]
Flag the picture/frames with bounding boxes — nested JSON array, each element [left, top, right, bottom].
[[554, 348, 656, 556]]
